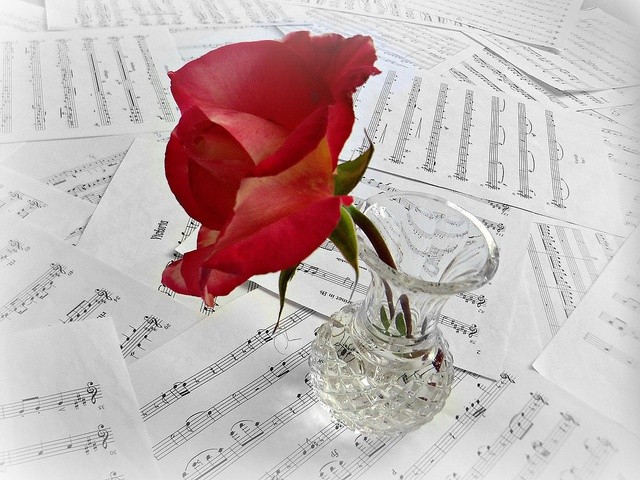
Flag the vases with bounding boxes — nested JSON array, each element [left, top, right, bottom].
[[307, 188, 501, 438]]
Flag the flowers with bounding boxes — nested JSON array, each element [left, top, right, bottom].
[[161, 30, 416, 332]]
